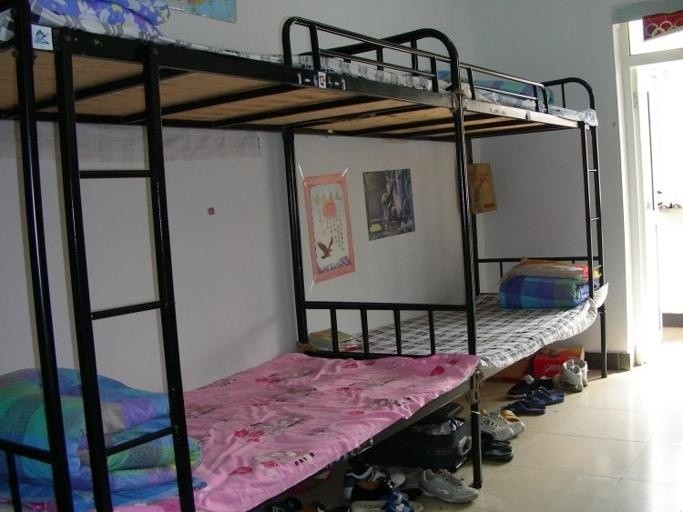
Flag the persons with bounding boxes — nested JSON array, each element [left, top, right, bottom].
[[382, 180, 396, 224]]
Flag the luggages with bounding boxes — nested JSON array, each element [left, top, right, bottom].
[[365, 403, 471, 472]]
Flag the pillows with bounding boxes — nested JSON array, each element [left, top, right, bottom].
[[2, 477, 208, 511], [498, 261, 593, 278]]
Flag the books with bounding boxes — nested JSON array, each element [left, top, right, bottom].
[[308, 327, 357, 350]]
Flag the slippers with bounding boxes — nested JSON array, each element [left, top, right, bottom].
[[503, 400, 544, 416], [525, 386, 566, 406], [480, 431, 514, 463]]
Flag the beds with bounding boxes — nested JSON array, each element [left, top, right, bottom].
[[1, 1, 482, 511], [294, 27, 609, 385]]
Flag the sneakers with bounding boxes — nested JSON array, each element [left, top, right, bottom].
[[348, 480, 424, 512], [552, 359, 583, 392], [341, 464, 409, 499], [419, 468, 479, 505], [481, 406, 524, 437], [505, 374, 555, 399], [479, 416, 516, 442], [575, 360, 588, 387]]
